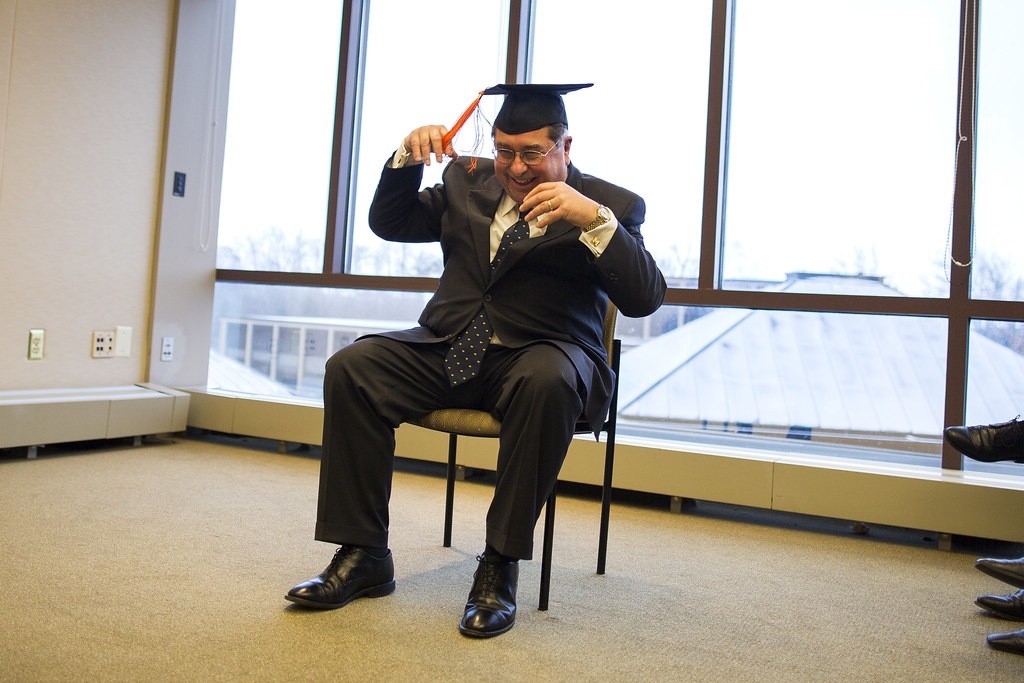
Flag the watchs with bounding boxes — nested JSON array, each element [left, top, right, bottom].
[[584, 204, 611, 231]]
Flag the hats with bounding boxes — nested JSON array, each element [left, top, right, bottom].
[[441, 81, 594, 171]]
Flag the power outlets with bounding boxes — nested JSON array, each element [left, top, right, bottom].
[[29, 329, 44, 361], [161, 336, 173, 362], [92, 330, 115, 359], [114, 326, 133, 357]]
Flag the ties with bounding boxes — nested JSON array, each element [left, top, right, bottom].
[[442, 207, 535, 386]]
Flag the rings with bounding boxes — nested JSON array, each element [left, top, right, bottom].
[[547, 199, 553, 210]]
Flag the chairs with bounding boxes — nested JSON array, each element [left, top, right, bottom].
[[405, 298, 622, 612]]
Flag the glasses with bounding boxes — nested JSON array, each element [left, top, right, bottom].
[[493, 133, 564, 166]]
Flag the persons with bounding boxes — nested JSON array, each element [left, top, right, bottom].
[[944, 419, 1024, 656], [284, 83, 668, 638]]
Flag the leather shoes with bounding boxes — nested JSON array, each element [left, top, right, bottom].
[[974, 588, 1024, 620], [460, 551, 519, 639], [986, 629, 1024, 653], [943, 416, 1024, 465], [975, 555, 1024, 590], [283, 544, 396, 608]]
